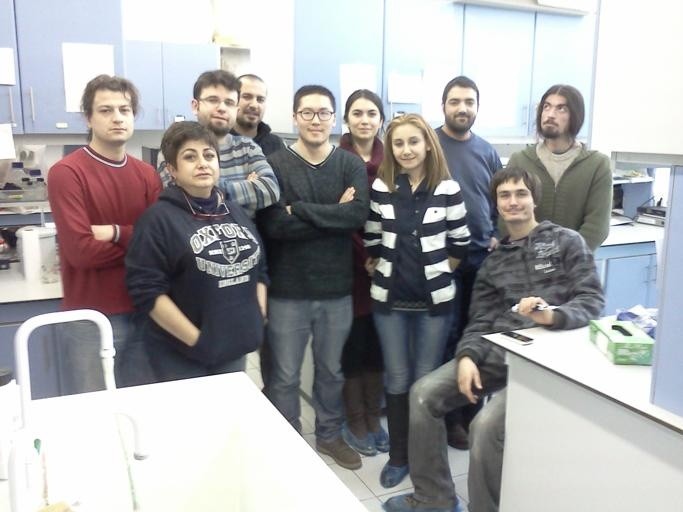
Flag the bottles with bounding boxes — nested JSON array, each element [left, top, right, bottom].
[[3, 161, 45, 200], [0, 368, 31, 479]]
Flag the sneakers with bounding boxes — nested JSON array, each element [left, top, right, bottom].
[[316, 436, 362, 471], [447, 424, 468, 450], [383, 493, 458, 512]]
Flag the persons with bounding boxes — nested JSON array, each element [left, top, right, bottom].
[[338, 88, 393, 455], [123, 121, 269, 382], [252, 85, 371, 470], [488, 83, 613, 253], [45, 74, 162, 394], [361, 114, 472, 489], [381, 168, 607, 512], [157, 70, 279, 219], [432, 75, 504, 451], [227, 74, 284, 157]]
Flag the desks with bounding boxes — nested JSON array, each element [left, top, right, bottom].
[[0, 370, 366, 512]]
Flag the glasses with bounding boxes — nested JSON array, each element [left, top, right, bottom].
[[296, 108, 334, 121], [182, 195, 231, 220], [197, 95, 239, 110]]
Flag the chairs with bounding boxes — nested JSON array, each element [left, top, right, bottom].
[[13, 308, 117, 401]]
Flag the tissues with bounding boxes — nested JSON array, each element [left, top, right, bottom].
[[587, 319, 654, 365]]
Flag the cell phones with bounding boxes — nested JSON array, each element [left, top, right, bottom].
[[501, 331, 535, 345]]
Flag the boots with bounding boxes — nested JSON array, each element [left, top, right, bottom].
[[344, 373, 376, 455], [380, 391, 408, 488], [364, 368, 390, 453]]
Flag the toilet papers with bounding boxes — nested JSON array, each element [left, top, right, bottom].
[[21, 229, 40, 279]]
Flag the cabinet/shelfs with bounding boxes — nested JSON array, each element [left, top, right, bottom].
[[124, 39, 217, 131], [0, 0, 123, 136], [0, 298, 73, 401], [592, 241, 658, 317], [464, 0, 590, 145], [294, 0, 464, 135]]
